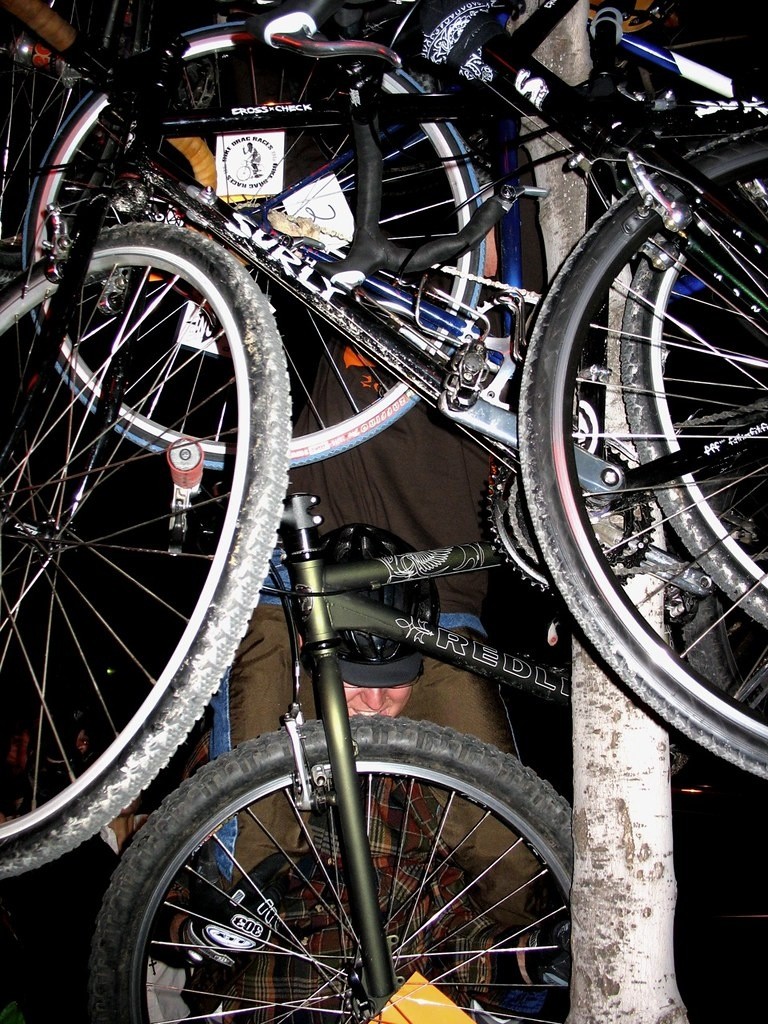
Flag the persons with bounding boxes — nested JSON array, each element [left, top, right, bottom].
[[86, 138, 571, 1024], [0, 704, 223, 1024]]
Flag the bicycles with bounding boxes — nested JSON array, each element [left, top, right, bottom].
[[0, 0, 768, 1024]]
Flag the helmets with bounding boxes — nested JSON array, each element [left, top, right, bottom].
[[301, 524, 443, 687]]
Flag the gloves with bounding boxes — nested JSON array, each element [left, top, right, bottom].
[[526, 919, 574, 988], [181, 853, 293, 968]]
